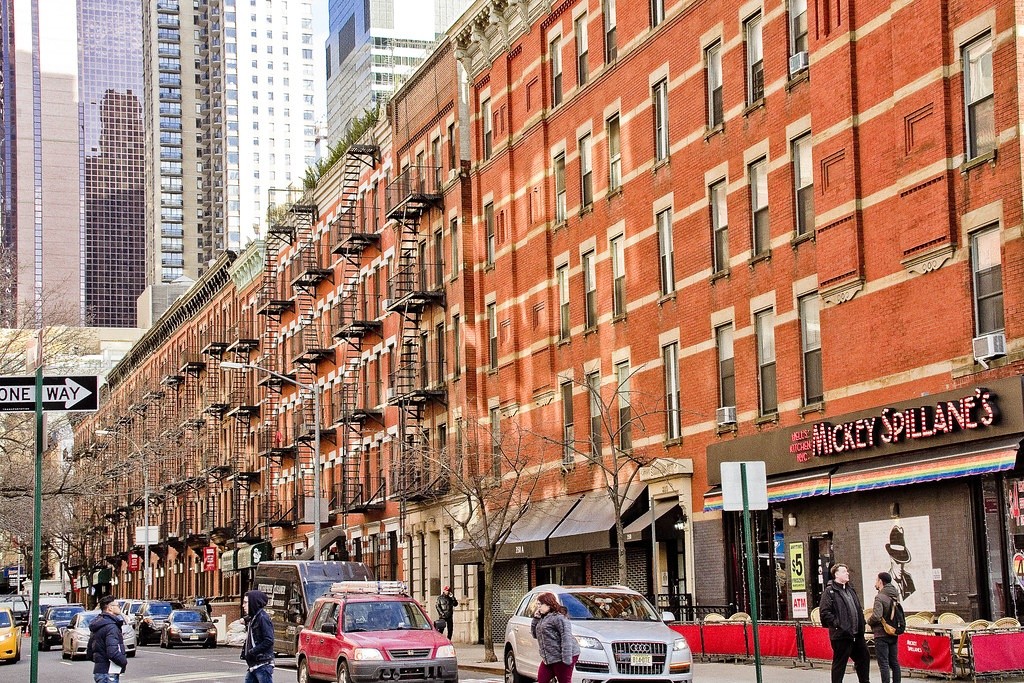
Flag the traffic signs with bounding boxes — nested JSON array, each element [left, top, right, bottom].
[[0, 373, 101, 414]]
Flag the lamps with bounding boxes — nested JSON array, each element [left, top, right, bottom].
[[789, 513, 797, 526], [674, 513, 688, 530], [890, 503, 899, 518]]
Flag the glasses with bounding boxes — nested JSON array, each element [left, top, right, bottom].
[[536, 602, 544, 608], [110, 604, 120, 608]]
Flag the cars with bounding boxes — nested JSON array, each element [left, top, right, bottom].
[[159, 609, 219, 649], [39, 598, 144, 652], [0, 606, 24, 664], [503, 583, 693, 683], [61, 611, 137, 661], [134, 601, 185, 646]]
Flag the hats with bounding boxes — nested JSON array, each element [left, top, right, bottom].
[[444, 585, 452, 592]]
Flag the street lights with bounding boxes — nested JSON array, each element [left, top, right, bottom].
[[218, 361, 321, 560], [94, 429, 150, 601]]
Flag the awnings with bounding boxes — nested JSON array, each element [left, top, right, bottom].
[[495, 493, 585, 559], [238, 541, 274, 569], [549, 481, 647, 554], [77, 571, 92, 589], [702, 436, 1024, 513], [623, 500, 680, 544], [450, 502, 527, 566], [93, 567, 112, 585], [221, 547, 241, 572]]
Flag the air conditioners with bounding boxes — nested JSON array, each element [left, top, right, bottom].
[[972, 334, 1007, 361], [789, 51, 809, 75], [381, 299, 393, 311], [448, 168, 456, 181], [387, 387, 394, 400], [716, 407, 736, 426]]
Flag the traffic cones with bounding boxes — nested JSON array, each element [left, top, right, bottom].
[[25, 626, 31, 638]]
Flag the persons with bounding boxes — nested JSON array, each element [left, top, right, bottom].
[[868, 572, 901, 683], [531, 593, 581, 683], [239, 590, 275, 683], [820, 563, 870, 683], [435, 586, 458, 641], [199, 598, 213, 618], [88, 595, 127, 683]]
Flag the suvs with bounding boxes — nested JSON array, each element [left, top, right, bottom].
[[294, 580, 459, 683]]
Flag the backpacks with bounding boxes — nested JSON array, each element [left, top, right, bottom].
[[880, 593, 906, 636]]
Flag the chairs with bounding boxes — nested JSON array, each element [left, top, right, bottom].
[[704, 613, 751, 664], [863, 607, 876, 658], [811, 606, 823, 626], [903, 611, 1021, 679]]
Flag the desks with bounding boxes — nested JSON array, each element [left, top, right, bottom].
[[917, 621, 993, 678]]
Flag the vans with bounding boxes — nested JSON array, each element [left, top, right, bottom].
[[250, 560, 377, 656], [28, 597, 68, 633]]
